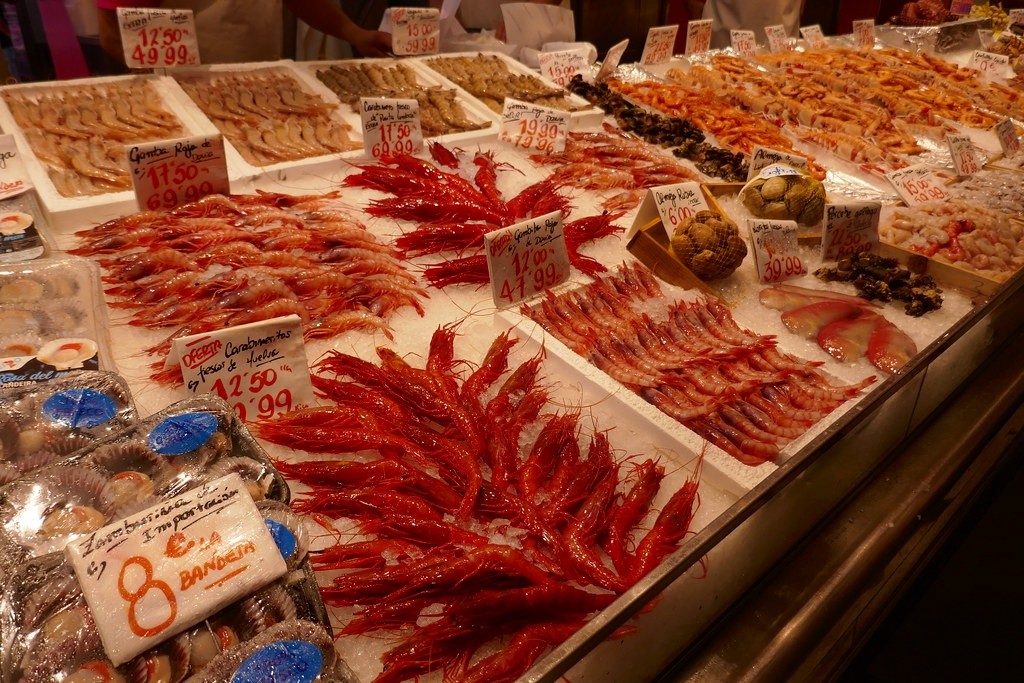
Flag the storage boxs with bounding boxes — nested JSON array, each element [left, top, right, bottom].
[[869, 131, 1024, 294], [0, 50, 604, 230], [495, 264, 876, 500]]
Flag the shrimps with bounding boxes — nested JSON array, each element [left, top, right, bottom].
[[0, 54, 696, 378], [604, 47, 1024, 182], [517, 260, 879, 469]]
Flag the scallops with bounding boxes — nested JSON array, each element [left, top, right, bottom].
[[0, 256, 354, 683]]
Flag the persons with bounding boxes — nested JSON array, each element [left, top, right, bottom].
[[687, 0, 803, 49], [97, 0, 403, 76]]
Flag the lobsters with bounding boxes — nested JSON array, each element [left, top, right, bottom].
[[244, 323, 693, 683]]
